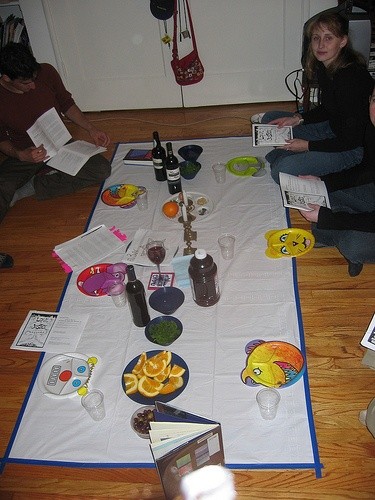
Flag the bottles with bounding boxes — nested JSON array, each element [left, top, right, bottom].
[[152, 131, 167, 182], [164, 142, 182, 195], [125, 265, 150, 327], [188, 249, 221, 307]]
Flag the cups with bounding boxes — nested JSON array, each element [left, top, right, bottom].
[[133, 188, 148, 211], [108, 279, 126, 307], [130, 406, 155, 439], [212, 163, 226, 184], [218, 234, 235, 260], [256, 388, 281, 420], [81, 389, 105, 421]]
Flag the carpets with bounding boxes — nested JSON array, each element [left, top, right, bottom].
[[3, 137, 324, 478]]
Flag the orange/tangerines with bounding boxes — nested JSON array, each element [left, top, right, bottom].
[[123, 350, 186, 398], [163, 201, 179, 217]]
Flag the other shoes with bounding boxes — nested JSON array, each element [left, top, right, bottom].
[[250, 113, 265, 123], [314, 242, 335, 247], [349, 263, 363, 276], [0, 253, 13, 268]]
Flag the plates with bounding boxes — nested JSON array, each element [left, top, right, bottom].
[[37, 352, 99, 399], [101, 184, 146, 208], [224, 156, 265, 176], [241, 340, 306, 389], [265, 227, 315, 258], [160, 192, 213, 223], [76, 263, 125, 298], [121, 350, 190, 405]]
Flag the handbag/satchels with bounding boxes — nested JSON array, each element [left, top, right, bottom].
[[171, 50, 204, 85]]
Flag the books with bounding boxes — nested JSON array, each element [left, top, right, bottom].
[[149, 401, 225, 500], [0, 14, 24, 44], [47, 140, 108, 177], [123, 149, 154, 166], [278, 172, 331, 211], [10, 311, 89, 353], [253, 124, 292, 146]]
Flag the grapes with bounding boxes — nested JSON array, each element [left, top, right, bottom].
[[134, 409, 154, 434]]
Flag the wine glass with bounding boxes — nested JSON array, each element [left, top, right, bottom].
[[146, 241, 169, 286]]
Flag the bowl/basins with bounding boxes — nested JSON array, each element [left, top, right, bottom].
[[178, 144, 204, 180], [144, 286, 185, 346]]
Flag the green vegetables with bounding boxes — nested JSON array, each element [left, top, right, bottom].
[[148, 319, 180, 344]]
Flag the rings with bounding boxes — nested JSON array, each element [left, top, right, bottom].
[[286, 148, 287, 150]]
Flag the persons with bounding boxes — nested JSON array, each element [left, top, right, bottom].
[[0, 41, 112, 268], [251, 12, 375, 185], [299, 89, 375, 277]]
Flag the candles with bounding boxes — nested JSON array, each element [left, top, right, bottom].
[[181, 203, 187, 222], [182, 190, 187, 206]]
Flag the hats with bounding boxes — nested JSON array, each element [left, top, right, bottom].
[[150, 0, 174, 20]]
